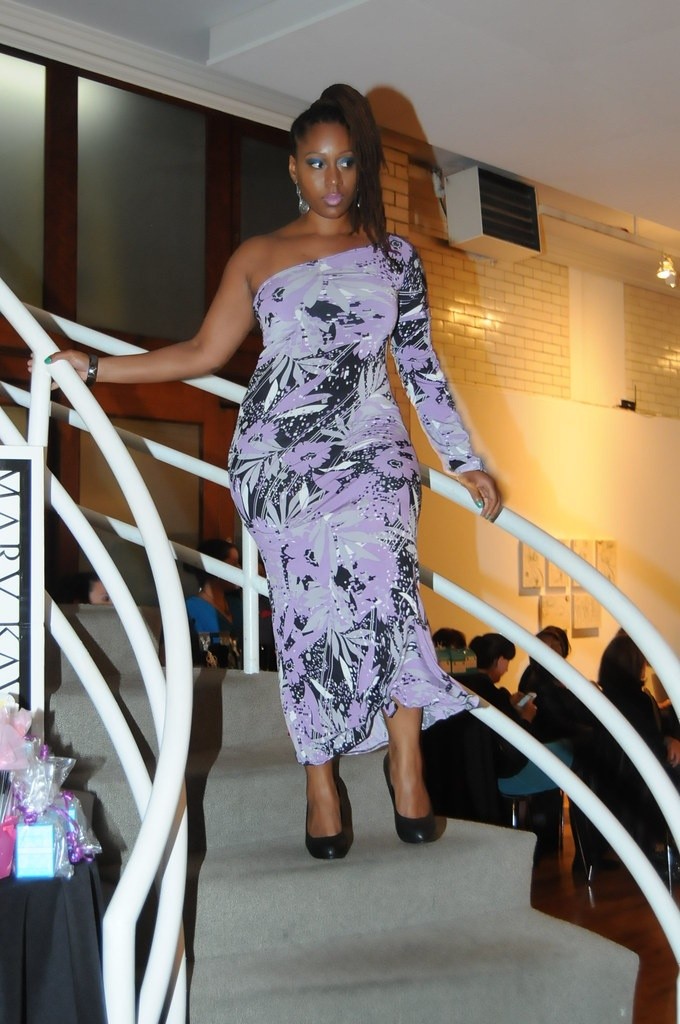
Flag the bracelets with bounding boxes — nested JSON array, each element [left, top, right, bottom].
[[86, 354, 98, 388]]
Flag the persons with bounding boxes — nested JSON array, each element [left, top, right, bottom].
[[424, 626, 680, 884], [27, 82, 502, 859]]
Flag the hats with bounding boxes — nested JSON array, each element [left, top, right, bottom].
[[536, 625, 567, 660]]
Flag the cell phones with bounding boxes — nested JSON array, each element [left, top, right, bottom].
[[517, 692, 538, 707]]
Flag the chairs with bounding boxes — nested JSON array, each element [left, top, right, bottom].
[[417, 672, 680, 893]]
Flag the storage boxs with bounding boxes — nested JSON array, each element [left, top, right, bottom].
[[14, 822, 68, 879]]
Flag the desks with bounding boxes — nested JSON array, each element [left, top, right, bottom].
[[0, 858, 107, 1024]]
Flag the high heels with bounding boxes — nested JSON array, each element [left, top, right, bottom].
[[382, 752, 437, 844], [304, 778, 349, 860]]
